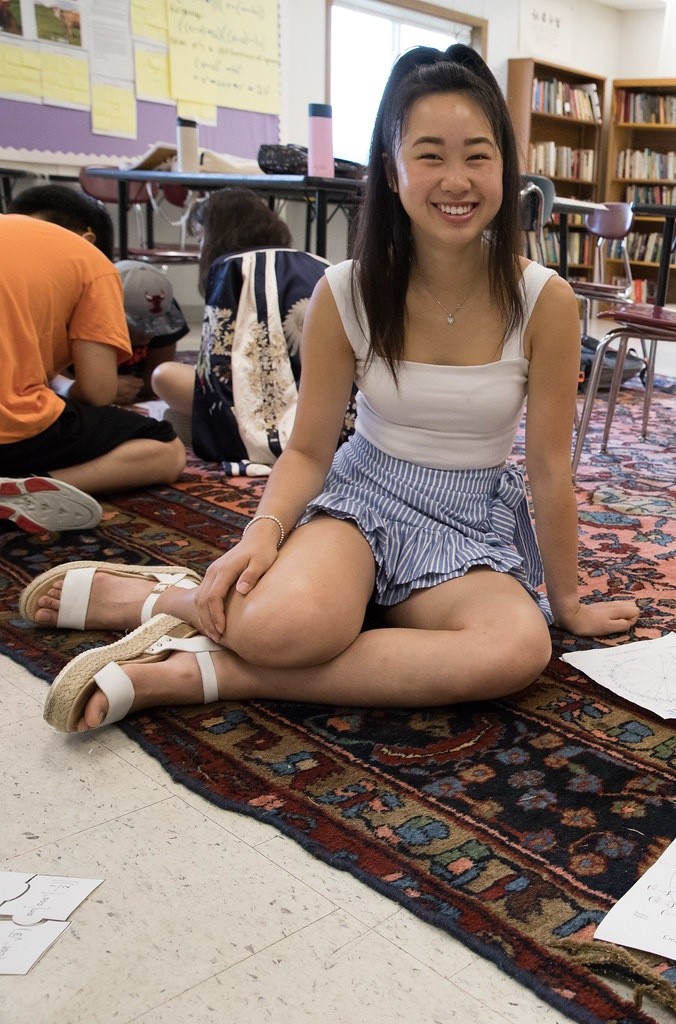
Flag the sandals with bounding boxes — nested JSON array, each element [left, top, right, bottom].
[[18, 560, 202, 635], [44, 613, 223, 735]]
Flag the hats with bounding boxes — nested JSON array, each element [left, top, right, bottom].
[[114, 260, 172, 337]]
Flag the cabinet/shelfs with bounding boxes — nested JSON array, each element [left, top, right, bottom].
[[506, 57, 676, 321]]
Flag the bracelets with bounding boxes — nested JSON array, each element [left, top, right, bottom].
[[241, 515, 286, 550]]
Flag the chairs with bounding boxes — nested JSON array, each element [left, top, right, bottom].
[[482, 173, 556, 268], [570, 302, 676, 475], [78, 166, 204, 269], [568, 201, 646, 359]]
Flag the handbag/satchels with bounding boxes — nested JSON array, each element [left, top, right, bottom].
[[143, 148, 256, 225], [257, 143, 366, 179]]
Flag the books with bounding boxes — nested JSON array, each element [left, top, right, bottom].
[[605, 229, 676, 265], [614, 148, 676, 182], [615, 88, 676, 124], [530, 73, 602, 123], [526, 140, 594, 185], [624, 184, 676, 217], [519, 226, 594, 266], [610, 275, 659, 310], [117, 140, 179, 172], [547, 193, 592, 226], [565, 275, 589, 301]]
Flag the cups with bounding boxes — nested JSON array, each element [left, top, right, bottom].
[[177, 117, 200, 173], [308, 104, 335, 178]]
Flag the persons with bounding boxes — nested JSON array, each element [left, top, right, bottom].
[[152, 187, 361, 469], [0, 184, 189, 532], [58, 258, 189, 405], [15, 42, 639, 735]]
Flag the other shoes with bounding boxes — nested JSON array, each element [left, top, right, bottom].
[[164, 409, 192, 443], [0, 478, 104, 533]]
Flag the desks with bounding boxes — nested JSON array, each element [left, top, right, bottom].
[[86, 169, 367, 260], [552, 198, 610, 281]]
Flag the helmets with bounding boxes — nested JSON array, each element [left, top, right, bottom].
[[578, 335, 642, 389]]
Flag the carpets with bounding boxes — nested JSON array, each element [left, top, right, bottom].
[[0, 350, 676, 1024]]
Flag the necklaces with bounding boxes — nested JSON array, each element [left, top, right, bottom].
[[416, 245, 487, 325]]
